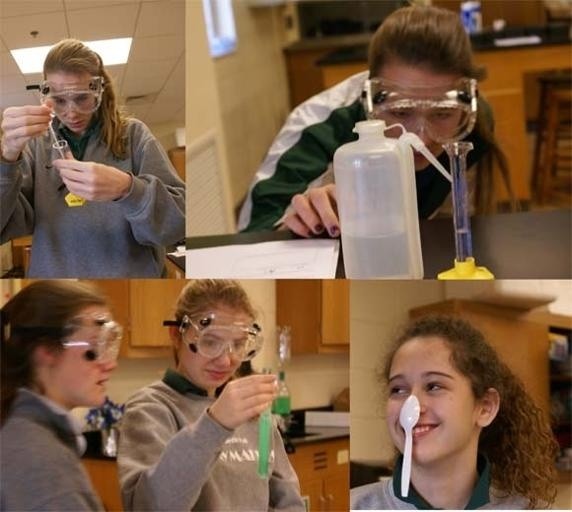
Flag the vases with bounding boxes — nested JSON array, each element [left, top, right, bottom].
[[100, 428, 119, 456]]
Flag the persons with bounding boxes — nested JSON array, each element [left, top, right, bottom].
[[238, 4, 523, 237], [118, 277, 307, 512], [1, 38, 186, 278], [0, 281, 123, 511], [351, 312, 561, 510]]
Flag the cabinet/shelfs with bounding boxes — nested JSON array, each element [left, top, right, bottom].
[[76, 279, 189, 359], [409, 298, 572, 488], [275, 279, 350, 355]]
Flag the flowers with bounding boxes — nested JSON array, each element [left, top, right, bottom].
[[84, 395, 125, 437]]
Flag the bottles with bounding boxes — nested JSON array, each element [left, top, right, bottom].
[[442, 141, 474, 263], [256, 367, 273, 480], [52, 140, 86, 207], [333, 119, 454, 279], [271, 372, 291, 434]]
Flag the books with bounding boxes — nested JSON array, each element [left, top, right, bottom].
[[187, 239, 340, 278]]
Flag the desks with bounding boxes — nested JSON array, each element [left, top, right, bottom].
[[82, 426, 350, 512], [315, 21, 572, 202], [187, 204, 572, 280]]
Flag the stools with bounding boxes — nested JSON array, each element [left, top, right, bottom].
[[531, 73, 572, 204]]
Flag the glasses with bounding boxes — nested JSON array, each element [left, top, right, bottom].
[[61, 314, 125, 366], [179, 311, 264, 364], [381, 75, 478, 150], [39, 75, 105, 118]]
